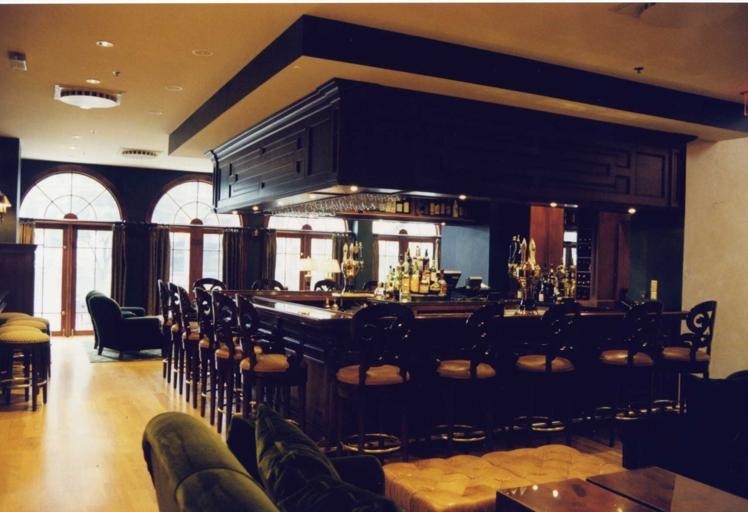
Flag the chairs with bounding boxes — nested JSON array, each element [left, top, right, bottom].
[[86, 290, 164, 359]]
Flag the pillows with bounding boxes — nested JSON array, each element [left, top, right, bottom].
[[271, 463, 399, 512], [254, 403, 340, 495], [228, 414, 264, 483], [683, 373, 747, 438]]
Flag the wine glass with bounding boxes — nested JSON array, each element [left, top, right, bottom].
[[269, 193, 388, 218]]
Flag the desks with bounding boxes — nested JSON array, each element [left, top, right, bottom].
[[587, 465, 748, 510], [495, 477, 656, 511]]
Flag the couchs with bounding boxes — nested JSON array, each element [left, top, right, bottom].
[[142, 411, 396, 511], [621, 370, 748, 500]]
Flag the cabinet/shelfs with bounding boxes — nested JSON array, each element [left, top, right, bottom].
[[332, 196, 466, 222], [574, 241, 592, 302]]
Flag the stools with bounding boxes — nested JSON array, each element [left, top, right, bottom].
[[0, 311, 50, 412], [383, 454, 533, 512], [482, 444, 623, 484]]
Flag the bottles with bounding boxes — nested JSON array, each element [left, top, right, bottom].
[[372, 246, 449, 303], [373, 194, 467, 218], [506, 234, 577, 304]]
